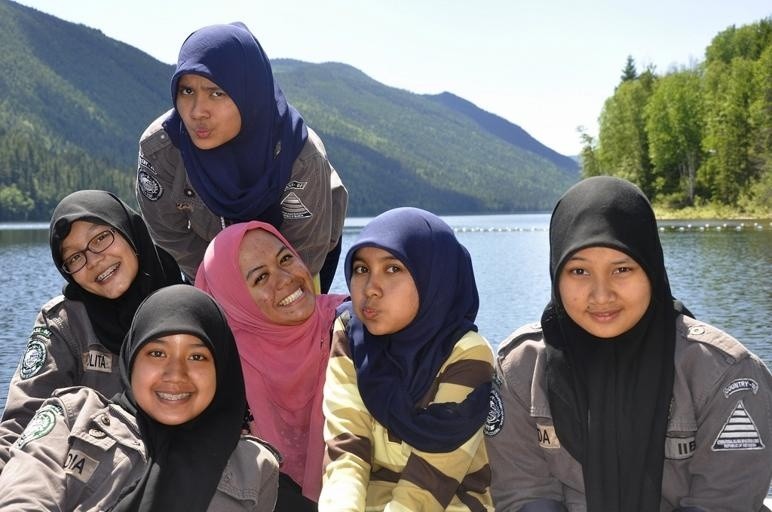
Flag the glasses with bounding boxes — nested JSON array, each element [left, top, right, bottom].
[[60, 229, 116, 277]]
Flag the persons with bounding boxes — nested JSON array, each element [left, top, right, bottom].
[[485, 176, 772, 512], [318, 206, 491, 512], [0, 284, 280, 512], [192, 220, 351, 512], [0, 190, 185, 465], [137, 22, 349, 295]]
